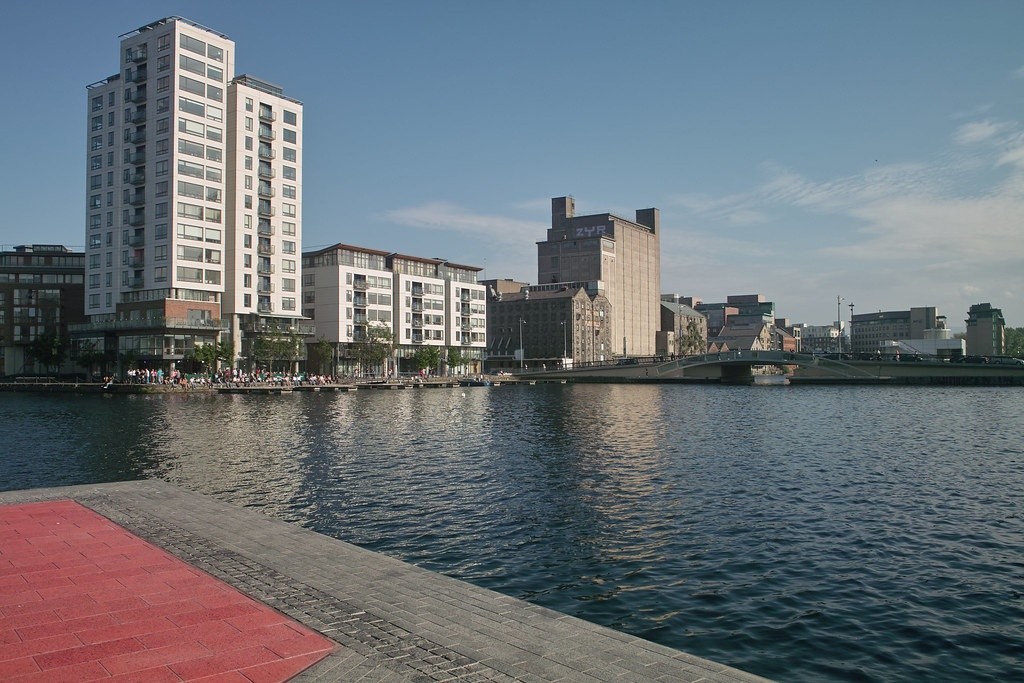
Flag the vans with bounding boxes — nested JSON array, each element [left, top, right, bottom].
[[949, 351, 1024, 365]]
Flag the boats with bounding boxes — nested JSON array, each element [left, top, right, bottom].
[[456, 378, 490, 386]]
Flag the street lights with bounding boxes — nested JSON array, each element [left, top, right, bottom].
[[837, 296, 845, 361], [519, 317, 527, 369], [561, 320, 567, 370], [849, 302, 854, 358]]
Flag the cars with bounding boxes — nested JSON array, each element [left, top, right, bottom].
[[900, 355, 923, 362]]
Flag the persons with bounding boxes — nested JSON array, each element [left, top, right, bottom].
[[873, 348, 919, 360], [100, 368, 332, 390], [354, 367, 369, 378], [388, 369, 392, 378], [419, 366, 429, 377], [499, 361, 562, 376]]
